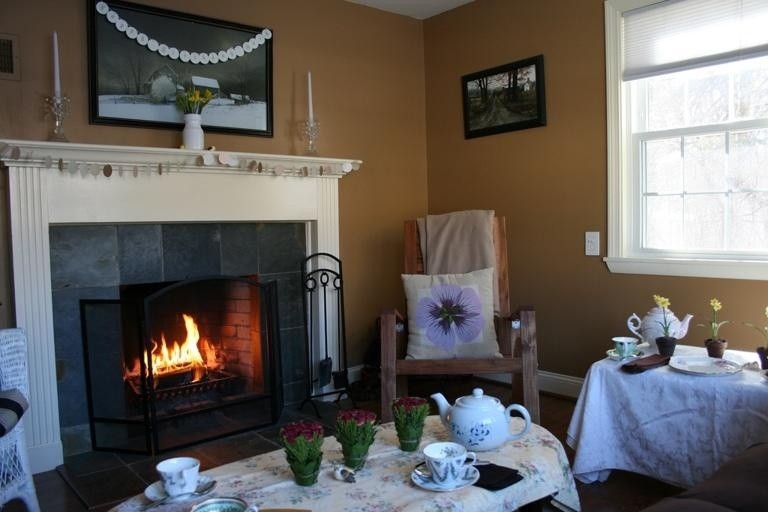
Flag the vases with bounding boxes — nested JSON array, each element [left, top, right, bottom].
[[703, 338, 727, 358], [756, 347, 768, 370], [396, 421, 426, 451], [656, 337, 677, 357], [337, 438, 375, 471], [287, 452, 323, 485], [183, 113, 204, 149]]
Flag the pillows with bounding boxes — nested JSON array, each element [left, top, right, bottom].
[[401, 267, 504, 361]]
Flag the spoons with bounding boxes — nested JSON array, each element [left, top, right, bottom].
[[142, 480, 218, 512]]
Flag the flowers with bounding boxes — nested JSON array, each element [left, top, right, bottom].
[[695, 297, 729, 342], [334, 409, 377, 443], [279, 421, 324, 465], [651, 294, 672, 337], [177, 86, 213, 115], [742, 305, 768, 349], [392, 397, 430, 430]]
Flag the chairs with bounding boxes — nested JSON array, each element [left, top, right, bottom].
[[380, 209, 540, 426], [0, 328, 40, 512]]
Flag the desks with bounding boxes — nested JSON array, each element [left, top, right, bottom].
[[565, 344, 768, 495], [106, 415, 581, 512]]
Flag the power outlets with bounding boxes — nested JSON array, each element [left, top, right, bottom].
[[585, 231, 600, 256]]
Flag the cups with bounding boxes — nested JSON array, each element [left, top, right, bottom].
[[189, 496, 258, 512], [422, 440, 477, 485], [156, 457, 200, 496]]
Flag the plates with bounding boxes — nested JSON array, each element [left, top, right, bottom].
[[606, 348, 644, 361], [411, 464, 480, 492], [669, 356, 743, 376], [144, 476, 218, 503]]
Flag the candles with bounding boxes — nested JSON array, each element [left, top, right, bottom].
[[51, 30, 62, 104], [307, 69, 314, 127]]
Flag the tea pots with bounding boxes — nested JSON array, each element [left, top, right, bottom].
[[430, 387, 531, 452], [626, 307, 695, 347]]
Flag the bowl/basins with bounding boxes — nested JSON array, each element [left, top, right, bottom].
[[612, 337, 639, 357]]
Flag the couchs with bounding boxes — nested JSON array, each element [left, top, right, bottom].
[[639, 442, 768, 512]]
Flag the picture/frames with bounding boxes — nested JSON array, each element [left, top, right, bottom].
[[461, 54, 547, 140], [0, 34, 20, 80], [87, 0, 274, 138]]
